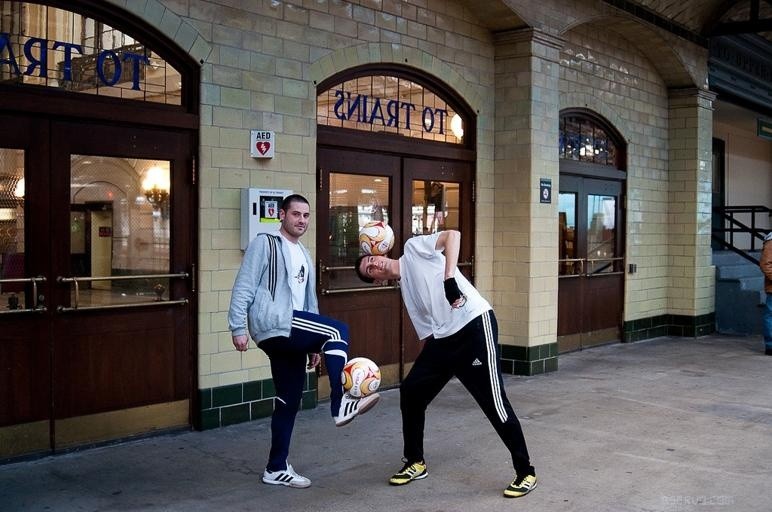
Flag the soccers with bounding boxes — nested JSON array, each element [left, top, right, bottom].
[[359, 221, 395, 256], [341, 356, 381, 397]]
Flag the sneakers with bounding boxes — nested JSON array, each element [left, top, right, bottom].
[[262, 460, 312, 488], [389, 457, 429, 486], [503, 465, 538, 498], [332, 390, 380, 427]]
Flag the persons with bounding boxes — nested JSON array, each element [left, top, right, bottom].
[[227, 193, 381, 489], [759, 231, 772, 356], [354, 229, 539, 498]]
